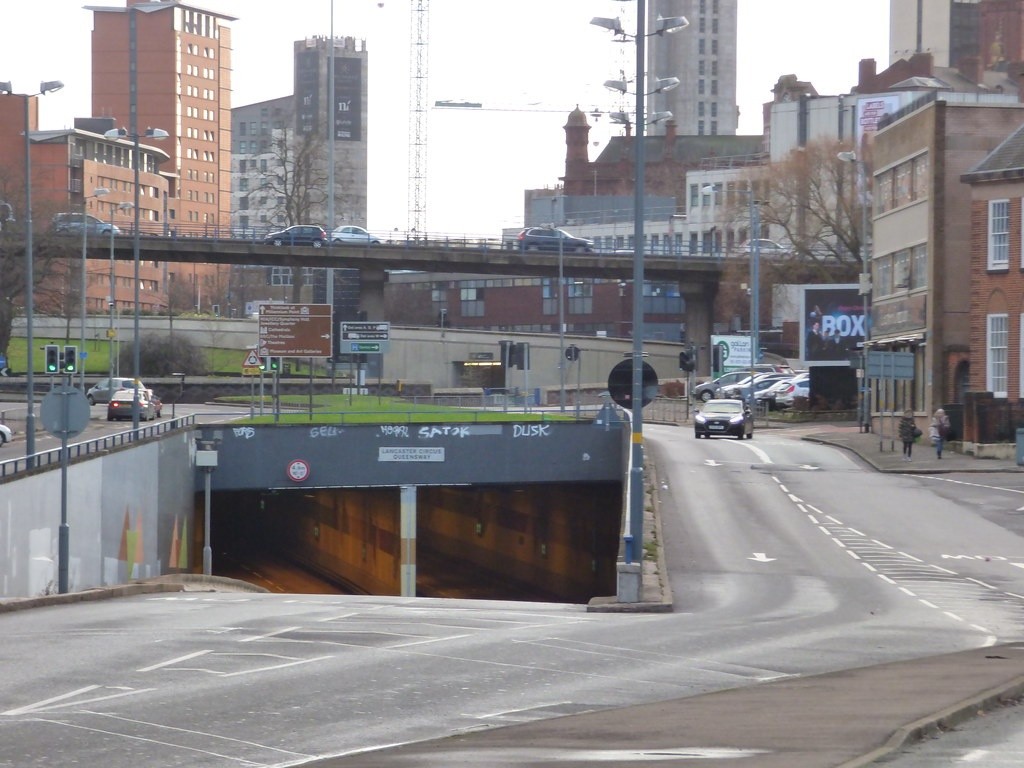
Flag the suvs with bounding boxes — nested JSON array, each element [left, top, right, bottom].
[[87, 377, 145, 406], [49, 212, 120, 236], [264, 225, 327, 248], [517, 226, 594, 252], [691, 364, 810, 411]]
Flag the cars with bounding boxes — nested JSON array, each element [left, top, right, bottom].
[[330, 226, 380, 244], [694, 399, 754, 440], [739, 239, 798, 260], [107, 389, 162, 421]]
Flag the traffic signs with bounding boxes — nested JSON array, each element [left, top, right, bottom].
[[340, 322, 391, 354], [258, 303, 333, 358]]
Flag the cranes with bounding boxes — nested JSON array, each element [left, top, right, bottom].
[[431, 99, 647, 149], [376, 0, 428, 246]]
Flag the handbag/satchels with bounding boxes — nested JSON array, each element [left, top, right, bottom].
[[913, 427, 922, 437], [938, 424, 947, 438]]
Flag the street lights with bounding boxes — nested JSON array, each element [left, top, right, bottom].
[[540, 223, 566, 412], [590, 0, 689, 587], [104, 126, 169, 439], [0, 81, 65, 468], [701, 185, 770, 413]]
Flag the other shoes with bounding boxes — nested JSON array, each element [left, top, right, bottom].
[[907, 457, 911, 461], [937, 452, 941, 458]]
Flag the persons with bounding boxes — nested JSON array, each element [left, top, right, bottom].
[[807, 322, 826, 360], [898, 409, 916, 461], [929, 409, 951, 459], [828, 330, 846, 359]]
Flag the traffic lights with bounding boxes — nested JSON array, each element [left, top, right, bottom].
[[45, 345, 78, 374], [271, 357, 279, 370], [259, 359, 267, 372]]
